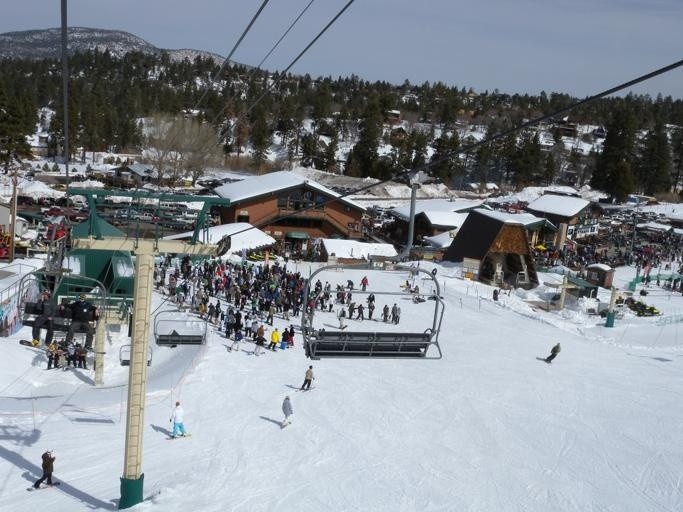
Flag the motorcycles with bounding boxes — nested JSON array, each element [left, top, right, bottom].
[[625, 296, 661, 317]]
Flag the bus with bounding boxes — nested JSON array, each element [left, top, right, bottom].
[[18, 212, 45, 226]]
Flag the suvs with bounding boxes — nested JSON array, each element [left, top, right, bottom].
[[248, 251, 279, 263]]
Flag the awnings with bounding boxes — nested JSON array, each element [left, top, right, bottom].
[[287, 233, 310, 238]]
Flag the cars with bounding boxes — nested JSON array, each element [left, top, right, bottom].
[[37, 197, 210, 241], [597, 209, 672, 232], [361, 205, 395, 229]]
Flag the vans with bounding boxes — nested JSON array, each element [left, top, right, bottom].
[[14, 197, 33, 205]]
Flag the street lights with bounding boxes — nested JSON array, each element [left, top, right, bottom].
[[406, 181, 421, 249]]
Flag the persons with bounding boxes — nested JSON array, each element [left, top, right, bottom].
[[546, 342, 561, 363], [533, 229, 682, 291], [280, 395, 293, 424], [29, 449, 60, 491], [167, 400, 190, 439], [31, 288, 100, 370], [302, 365, 317, 390], [153, 241, 437, 353]]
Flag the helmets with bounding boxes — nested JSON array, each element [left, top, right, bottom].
[[47, 449, 53, 455]]
